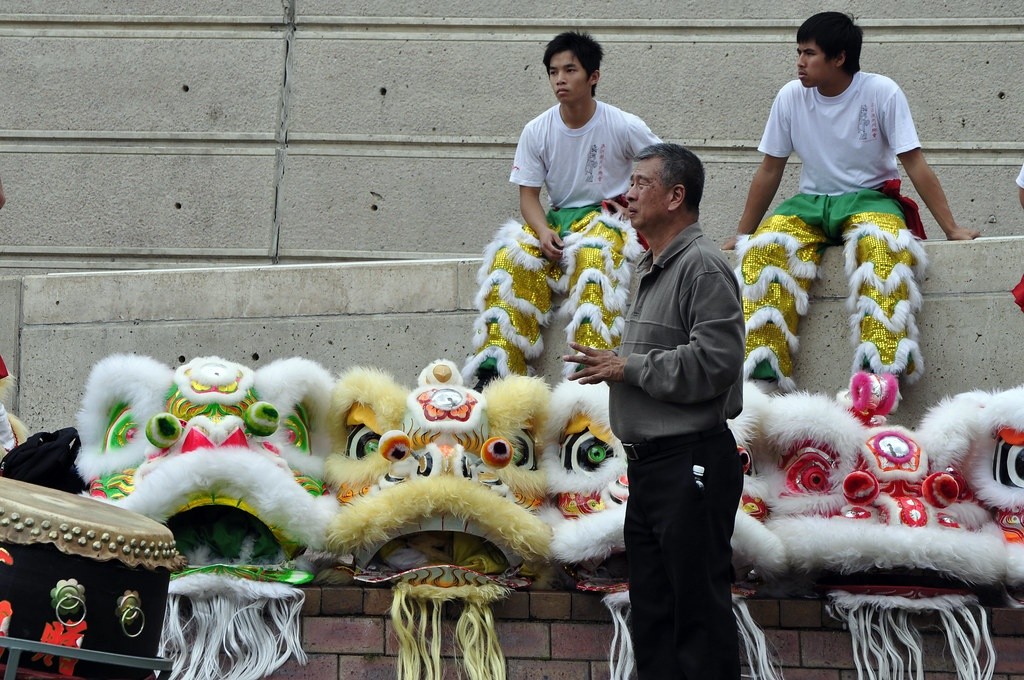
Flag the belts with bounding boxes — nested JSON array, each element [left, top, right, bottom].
[[621, 427, 726, 461]]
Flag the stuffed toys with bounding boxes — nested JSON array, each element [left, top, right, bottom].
[[538, 373, 632, 680], [756, 385, 900, 680], [714, 381, 779, 680], [73, 357, 339, 680], [834, 372, 1004, 680], [323, 358, 552, 680], [909, 381, 1024, 680]]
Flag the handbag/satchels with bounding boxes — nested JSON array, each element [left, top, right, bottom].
[[3, 427, 85, 495]]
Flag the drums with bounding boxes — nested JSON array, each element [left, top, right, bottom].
[[0, 476, 188, 680]]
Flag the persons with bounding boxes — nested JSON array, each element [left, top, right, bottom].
[[473, 29, 663, 383], [561, 144, 751, 678], [720, 9, 984, 415]]
